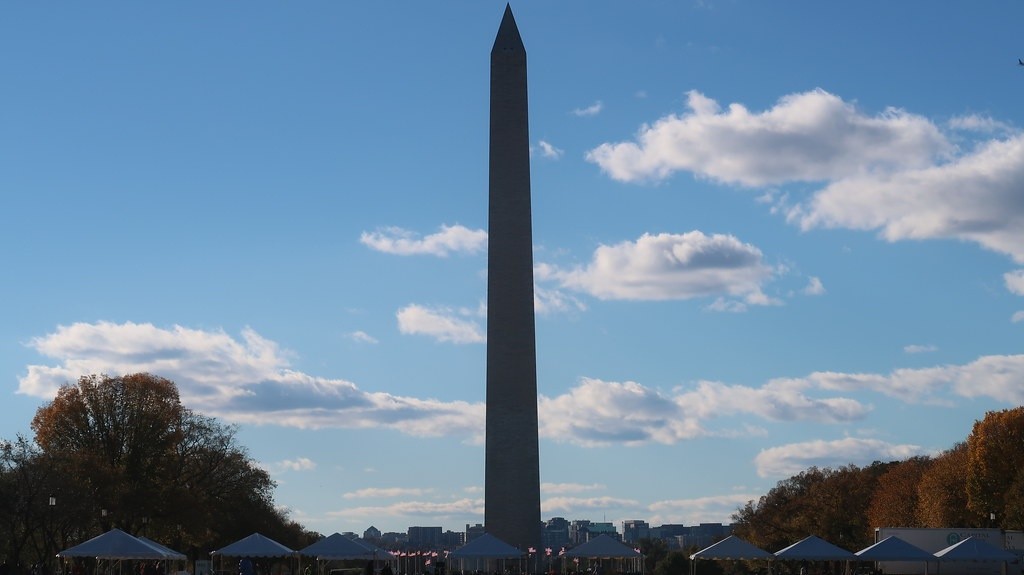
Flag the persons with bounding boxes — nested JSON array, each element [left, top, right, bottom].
[[176, 564, 190, 575], [590, 562, 603, 575], [800, 559, 809, 575], [770, 560, 778, 575]]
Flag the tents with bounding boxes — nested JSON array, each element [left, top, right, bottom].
[[768, 535, 857, 575], [933, 536, 1021, 575], [447, 533, 528, 575], [208, 532, 295, 575], [297, 533, 398, 575], [57, 528, 188, 575], [689, 534, 775, 575], [562, 534, 648, 575], [851, 536, 939, 575]]
